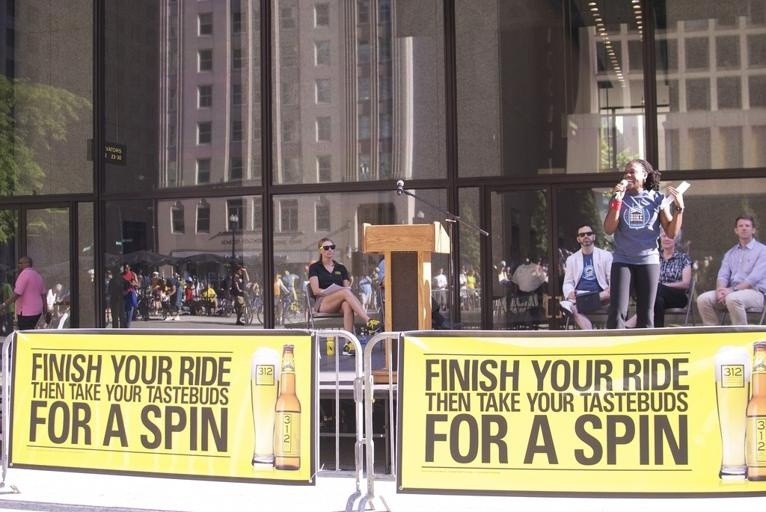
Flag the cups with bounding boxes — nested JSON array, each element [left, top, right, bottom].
[[715, 346, 750, 480], [250, 348, 279, 469]]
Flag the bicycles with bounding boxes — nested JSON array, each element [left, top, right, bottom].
[[257, 296, 307, 327], [241, 293, 254, 327]]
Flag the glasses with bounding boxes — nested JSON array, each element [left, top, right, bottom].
[[577, 232, 593, 237], [320, 245, 335, 250], [16, 262, 22, 265]]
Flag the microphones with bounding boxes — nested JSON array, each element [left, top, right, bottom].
[[612, 178, 629, 201], [396, 179, 406, 195]]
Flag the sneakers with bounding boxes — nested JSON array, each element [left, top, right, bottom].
[[626, 314, 638, 328], [365, 319, 382, 335], [342, 341, 357, 356], [557, 300, 573, 315]]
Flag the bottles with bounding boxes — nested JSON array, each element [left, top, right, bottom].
[[326, 337, 334, 355], [746, 341, 765, 481], [274, 344, 301, 471]]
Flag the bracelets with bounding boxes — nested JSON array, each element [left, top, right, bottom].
[[610, 198, 622, 212], [675, 207, 686, 212]]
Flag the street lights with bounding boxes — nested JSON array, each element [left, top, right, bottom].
[[225, 213, 240, 317]]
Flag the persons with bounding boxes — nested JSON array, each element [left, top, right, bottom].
[[308, 238, 384, 356], [273, 273, 291, 326], [282, 270, 302, 314], [231, 265, 250, 326], [1, 256, 52, 330], [603, 159, 685, 328], [44, 283, 71, 329], [696, 214, 766, 325], [122, 264, 138, 323], [431, 255, 552, 317], [558, 249, 572, 276], [623, 224, 694, 328], [108, 267, 132, 327], [138, 271, 230, 321], [558, 223, 613, 330], [370, 269, 379, 311], [358, 272, 372, 313], [1, 265, 16, 335]]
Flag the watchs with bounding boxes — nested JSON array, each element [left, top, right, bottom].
[[2, 303, 7, 307], [733, 284, 738, 292]]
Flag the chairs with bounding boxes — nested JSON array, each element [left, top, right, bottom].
[[305, 283, 356, 358], [665, 271, 698, 327], [715, 302, 766, 324]]
